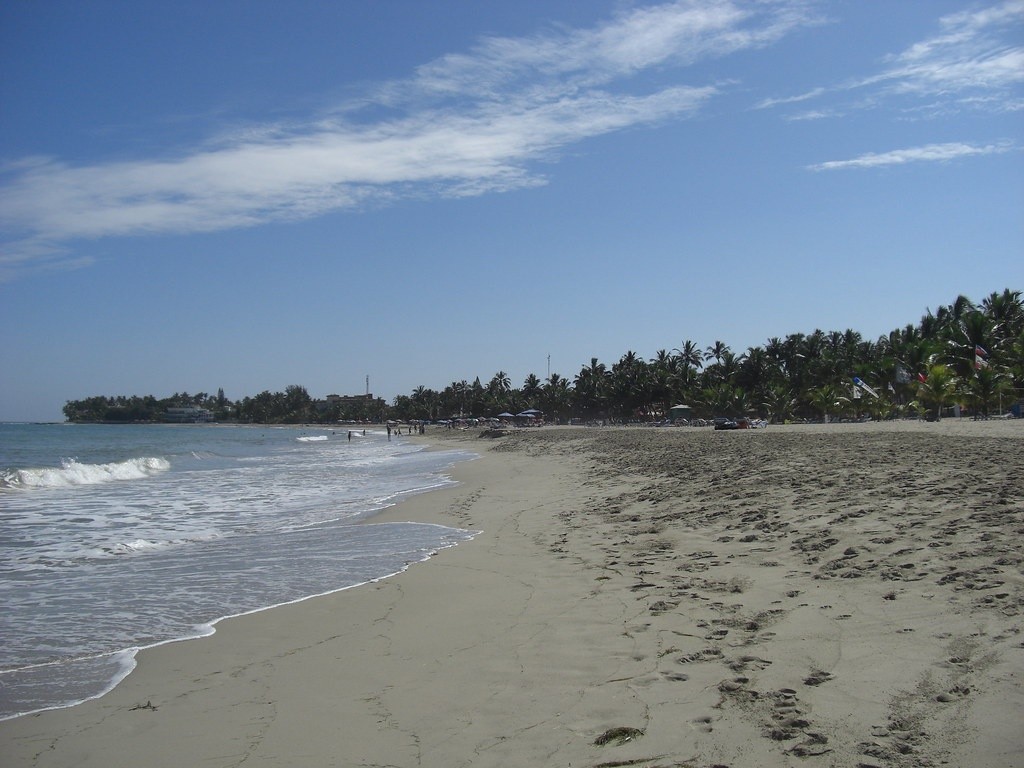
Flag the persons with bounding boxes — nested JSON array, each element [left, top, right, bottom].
[[587, 419, 710, 430], [747, 417, 750, 428], [347, 419, 542, 444]]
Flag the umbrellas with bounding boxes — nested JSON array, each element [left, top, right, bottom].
[[339, 409, 542, 423], [671, 404, 692, 411]]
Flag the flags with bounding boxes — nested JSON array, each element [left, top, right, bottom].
[[919, 373, 926, 381], [975, 345, 990, 370]]
